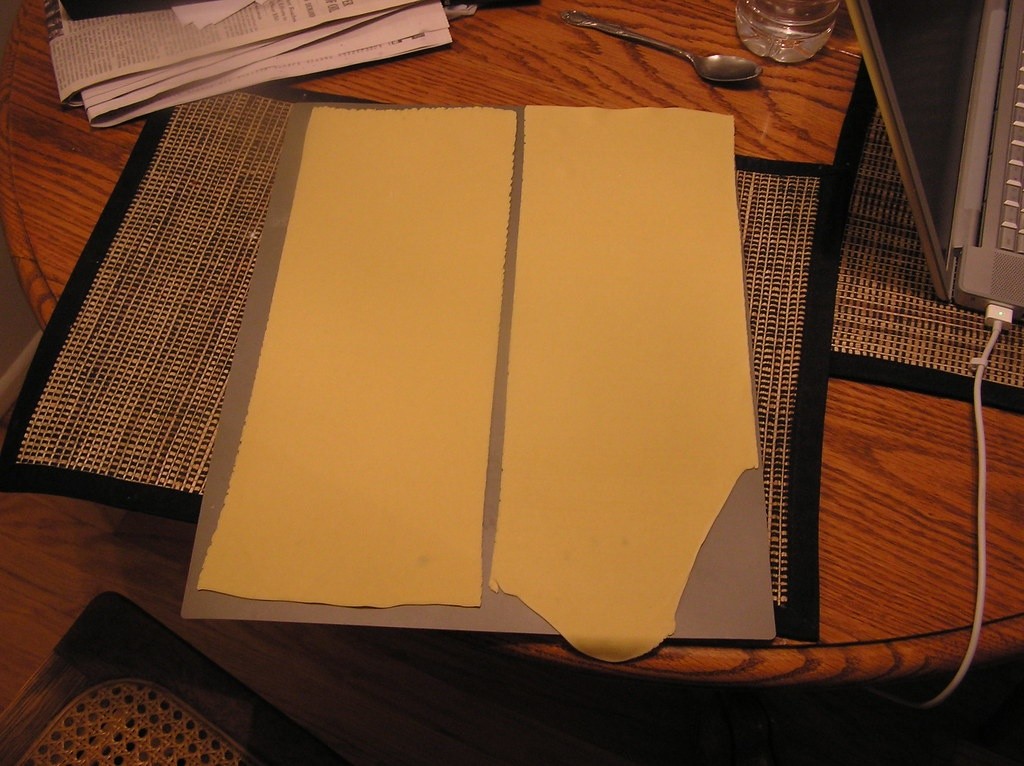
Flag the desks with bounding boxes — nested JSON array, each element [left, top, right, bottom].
[[0, 0, 1024, 680]]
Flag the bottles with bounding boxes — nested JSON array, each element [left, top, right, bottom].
[[735, 0, 841, 63]]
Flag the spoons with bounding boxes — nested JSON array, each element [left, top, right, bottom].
[[561, 10, 761, 82]]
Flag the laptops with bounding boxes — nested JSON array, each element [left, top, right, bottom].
[[845, 0, 1024, 320]]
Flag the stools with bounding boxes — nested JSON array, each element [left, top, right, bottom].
[[0, 590, 354, 766]]
